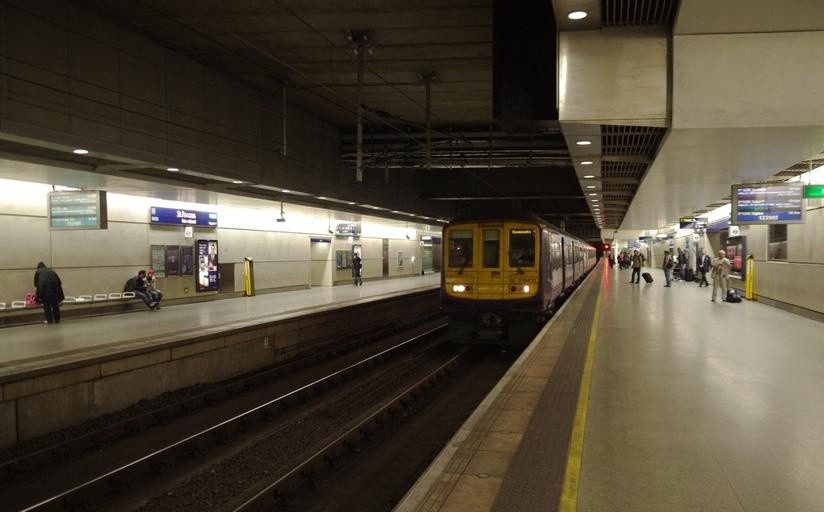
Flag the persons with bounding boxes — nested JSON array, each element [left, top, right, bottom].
[[697, 249, 711, 287], [617, 249, 645, 284], [125, 269, 159, 312], [33, 261, 65, 323], [142, 269, 163, 310], [662, 250, 673, 287], [710, 249, 732, 302], [352, 253, 363, 286], [207, 246, 218, 270], [608, 252, 614, 268], [676, 247, 687, 282]]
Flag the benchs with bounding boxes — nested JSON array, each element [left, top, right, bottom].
[[0, 291, 142, 316]]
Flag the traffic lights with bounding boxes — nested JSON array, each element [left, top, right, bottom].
[[602, 243, 610, 251]]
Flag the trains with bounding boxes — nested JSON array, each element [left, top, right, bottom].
[[440, 208, 596, 345]]
